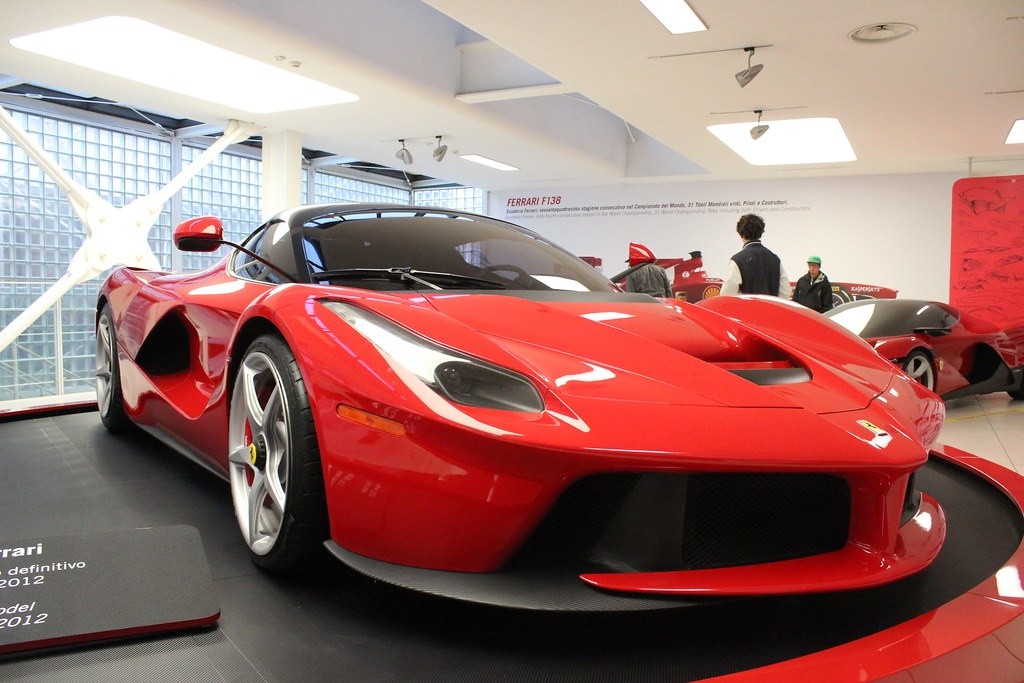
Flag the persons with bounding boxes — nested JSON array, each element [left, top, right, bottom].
[[720, 213, 792, 298], [626, 259, 673, 298], [792, 255, 832, 314]]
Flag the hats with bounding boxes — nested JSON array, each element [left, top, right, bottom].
[[807, 256, 821, 265]]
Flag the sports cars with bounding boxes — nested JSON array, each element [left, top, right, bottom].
[[608, 259, 897, 313], [95, 201, 948, 611], [821, 299, 1024, 402]]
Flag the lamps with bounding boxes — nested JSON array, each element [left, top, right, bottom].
[[735, 47, 764, 89], [394, 140, 414, 166], [750, 110, 769, 141], [433, 135, 448, 163]]
